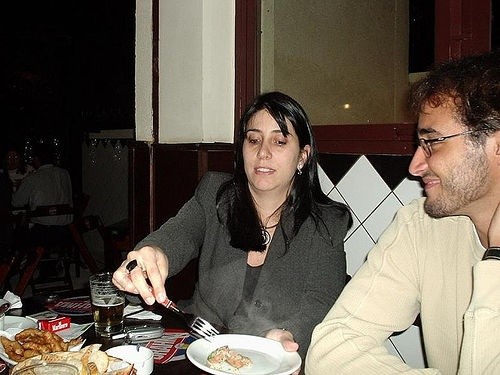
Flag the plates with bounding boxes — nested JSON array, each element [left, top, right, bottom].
[[4, 315, 38, 333], [186, 333, 302, 375]]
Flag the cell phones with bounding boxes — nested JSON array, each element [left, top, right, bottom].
[[112, 324, 165, 340]]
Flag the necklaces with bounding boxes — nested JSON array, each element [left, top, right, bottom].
[[260, 223, 279, 246]]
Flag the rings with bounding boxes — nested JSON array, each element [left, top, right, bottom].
[[125, 259, 142, 275]]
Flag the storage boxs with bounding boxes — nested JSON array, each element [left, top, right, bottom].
[[26, 309, 71, 333], [24, 257, 63, 276], [103, 238, 132, 273]]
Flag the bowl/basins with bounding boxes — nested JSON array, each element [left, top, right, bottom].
[[0, 352, 18, 369], [10, 351, 134, 375], [0, 313, 5, 331]]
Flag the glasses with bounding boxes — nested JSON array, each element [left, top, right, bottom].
[[419, 128, 494, 158]]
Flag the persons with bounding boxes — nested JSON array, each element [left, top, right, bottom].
[[0, 148, 76, 277], [113, 89, 351, 375], [303, 53, 500, 375]]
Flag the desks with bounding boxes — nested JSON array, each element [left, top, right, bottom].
[[0, 294, 225, 375]]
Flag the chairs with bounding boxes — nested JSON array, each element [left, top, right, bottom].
[[0, 194, 106, 295]]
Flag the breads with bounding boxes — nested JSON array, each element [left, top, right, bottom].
[[12, 344, 135, 375]]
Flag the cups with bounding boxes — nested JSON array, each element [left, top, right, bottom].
[[89, 271, 125, 338]]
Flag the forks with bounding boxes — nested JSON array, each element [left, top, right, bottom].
[[148, 285, 219, 342]]
